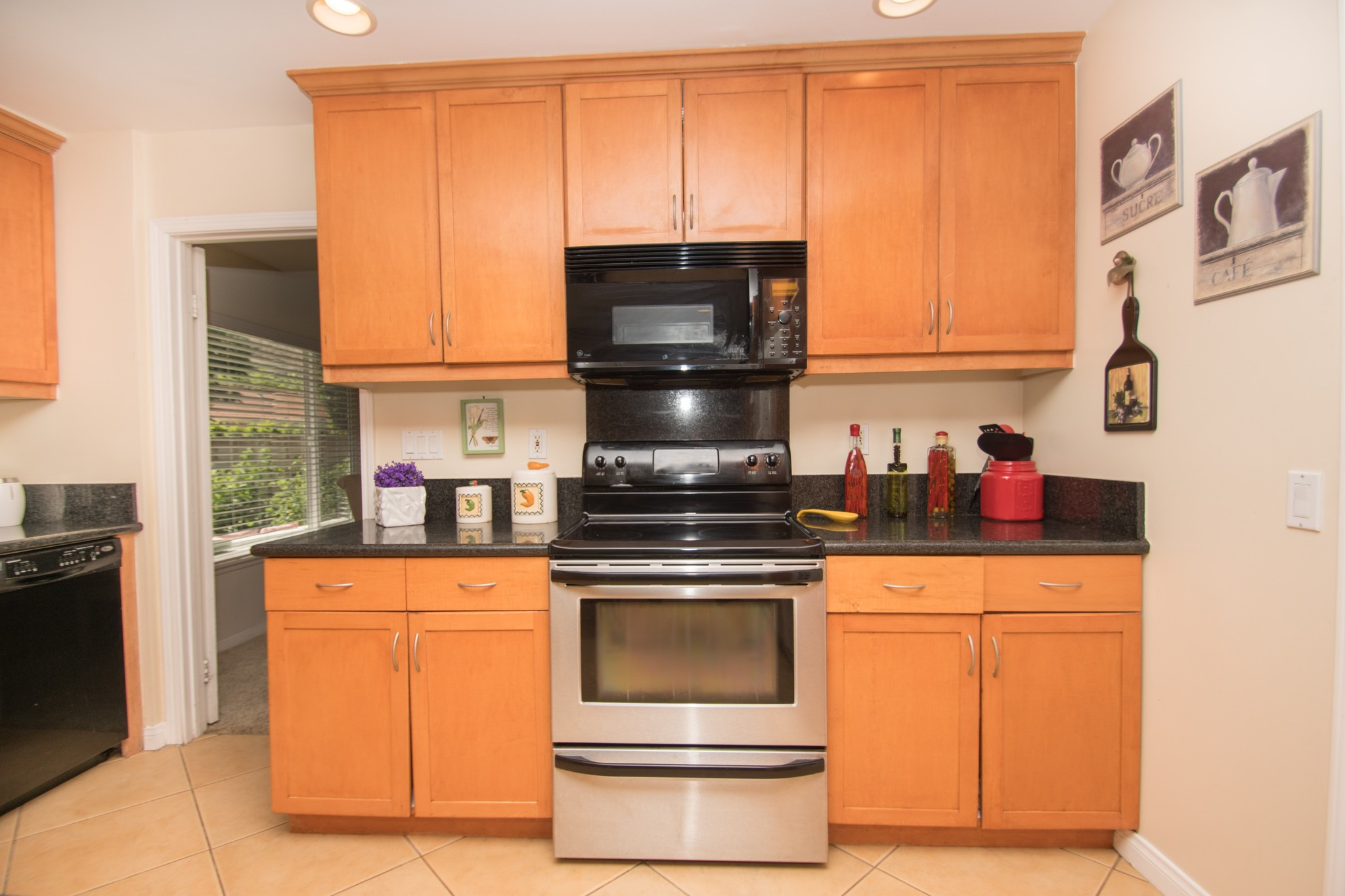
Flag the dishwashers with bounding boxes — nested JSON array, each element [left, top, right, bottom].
[[0, 537, 129, 807]]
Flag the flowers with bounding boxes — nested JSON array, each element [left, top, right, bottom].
[[373, 460, 426, 488]]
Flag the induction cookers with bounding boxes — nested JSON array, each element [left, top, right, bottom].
[[549, 440, 825, 559]]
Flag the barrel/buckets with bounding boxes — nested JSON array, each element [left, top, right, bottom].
[[337, 474, 362, 522]]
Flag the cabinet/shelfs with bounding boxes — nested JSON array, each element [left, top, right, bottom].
[[824, 552, 1142, 850], [561, 42, 805, 248], [0, 106, 67, 400], [804, 30, 1089, 388], [284, 56, 572, 392], [263, 557, 554, 839]]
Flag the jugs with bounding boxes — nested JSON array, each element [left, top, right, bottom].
[[1215, 155, 1288, 249]]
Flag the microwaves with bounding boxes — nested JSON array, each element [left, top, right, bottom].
[[564, 241, 807, 388]]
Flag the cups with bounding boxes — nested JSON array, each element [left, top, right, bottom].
[[1111, 133, 1163, 193]]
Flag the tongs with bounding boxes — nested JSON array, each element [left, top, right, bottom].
[[970, 455, 998, 510]]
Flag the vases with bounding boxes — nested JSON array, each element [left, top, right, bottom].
[[373, 485, 427, 528]]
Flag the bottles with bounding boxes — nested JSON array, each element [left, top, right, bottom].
[[456, 481, 492, 525], [25, 485, 66, 523], [888, 428, 908, 518], [980, 460, 1045, 521], [511, 461, 558, 525], [0, 478, 25, 526], [927, 432, 956, 520], [844, 424, 869, 519]]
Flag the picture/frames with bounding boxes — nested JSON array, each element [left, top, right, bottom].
[[1193, 109, 1324, 306], [1096, 79, 1184, 246]]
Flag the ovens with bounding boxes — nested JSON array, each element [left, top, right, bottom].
[[549, 560, 829, 864]]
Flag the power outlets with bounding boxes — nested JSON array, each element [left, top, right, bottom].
[[850, 424, 870, 455], [528, 428, 547, 459]]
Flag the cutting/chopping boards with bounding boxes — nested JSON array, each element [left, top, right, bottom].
[[1103, 296, 1156, 430]]
